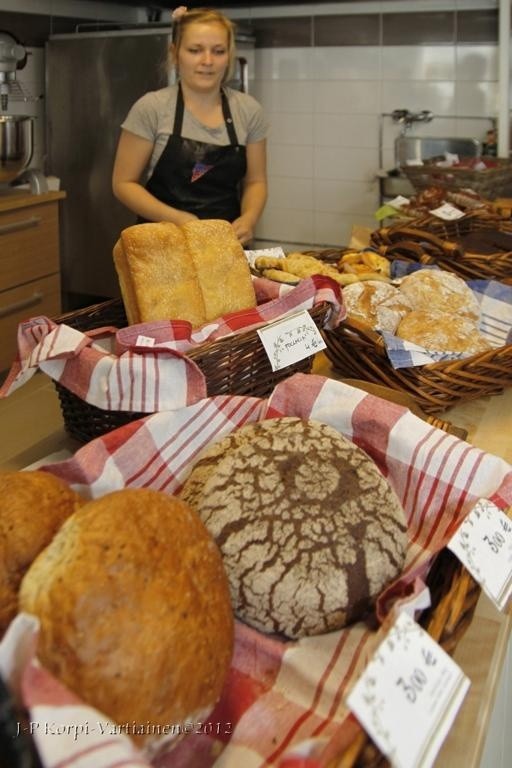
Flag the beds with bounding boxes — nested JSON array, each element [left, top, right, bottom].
[[1, 330, 511, 768]]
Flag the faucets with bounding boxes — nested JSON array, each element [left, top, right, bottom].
[[391, 109, 433, 138]]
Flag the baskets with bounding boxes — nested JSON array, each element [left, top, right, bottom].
[[0, 379, 481, 767], [368, 209, 512, 281], [320, 278, 512, 415], [250, 240, 437, 295], [40, 267, 330, 440]]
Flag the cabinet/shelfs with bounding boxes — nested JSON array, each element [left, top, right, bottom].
[[0, 192, 65, 374]]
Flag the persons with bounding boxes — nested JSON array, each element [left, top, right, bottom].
[[113, 8, 267, 248]]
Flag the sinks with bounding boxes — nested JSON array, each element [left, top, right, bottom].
[[373, 137, 482, 198]]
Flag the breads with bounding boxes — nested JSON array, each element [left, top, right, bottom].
[[17, 487, 234, 760], [1, 468, 89, 643], [445, 229, 512, 254], [173, 418, 409, 640], [399, 269, 483, 320], [396, 308, 493, 353], [257, 250, 391, 285], [112, 221, 206, 328], [185, 219, 256, 321], [342, 280, 409, 334], [411, 186, 511, 222]]
